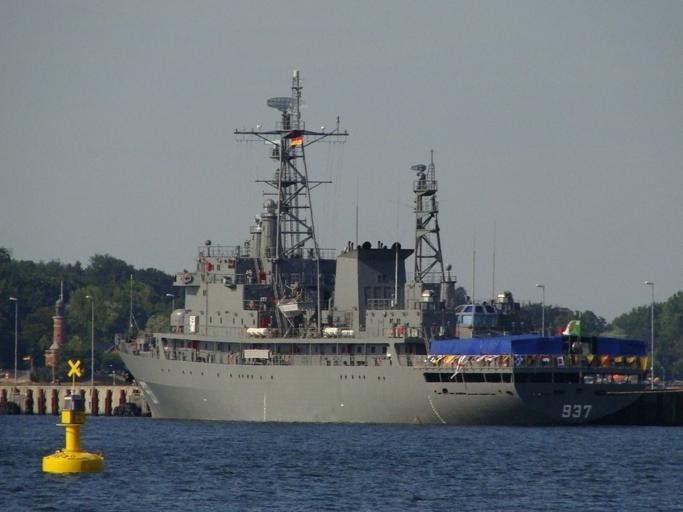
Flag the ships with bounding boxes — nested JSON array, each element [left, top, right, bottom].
[[104, 67, 649, 429]]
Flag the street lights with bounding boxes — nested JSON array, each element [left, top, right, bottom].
[[9, 296, 19, 380], [643, 280, 655, 392], [533, 283, 545, 338], [85, 295, 95, 384]]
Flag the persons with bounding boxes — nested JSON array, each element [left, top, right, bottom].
[[245, 267, 253, 283]]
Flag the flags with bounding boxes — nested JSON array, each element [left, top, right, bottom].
[[449, 356, 466, 380], [288, 130, 303, 146], [22, 354, 32, 361], [557, 319, 581, 338], [422, 351, 650, 372]]
